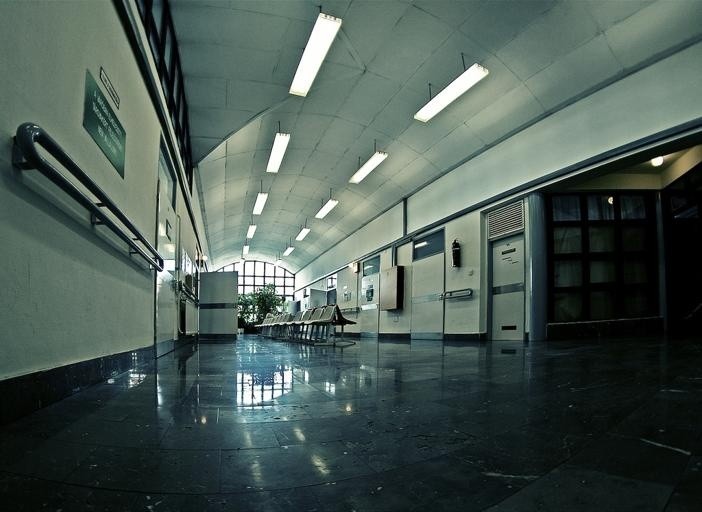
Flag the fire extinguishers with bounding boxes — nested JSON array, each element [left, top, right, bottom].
[[452, 238, 462, 266]]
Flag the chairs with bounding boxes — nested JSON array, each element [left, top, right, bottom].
[[254, 303, 357, 346]]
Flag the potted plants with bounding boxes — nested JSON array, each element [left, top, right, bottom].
[[236, 293, 259, 334]]
